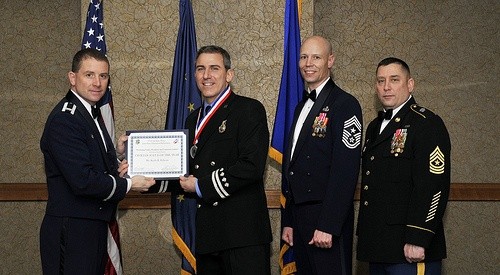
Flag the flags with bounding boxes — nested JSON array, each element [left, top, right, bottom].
[[164, 0, 202, 275], [80, 0, 124, 275], [267, 0, 306, 275]]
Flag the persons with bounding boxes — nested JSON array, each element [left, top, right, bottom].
[[39, 48, 156, 275], [283, 36, 363, 275], [357, 57, 451, 275], [117, 45, 273, 275]]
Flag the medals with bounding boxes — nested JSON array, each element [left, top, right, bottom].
[[190, 144, 197, 159]]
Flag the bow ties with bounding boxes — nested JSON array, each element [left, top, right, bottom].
[[302, 89, 316, 102], [91, 104, 101, 119], [378, 108, 393, 122]]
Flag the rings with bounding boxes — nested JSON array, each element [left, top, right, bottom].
[[323, 245, 328, 247]]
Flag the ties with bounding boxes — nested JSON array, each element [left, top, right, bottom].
[[205, 106, 212, 117]]
